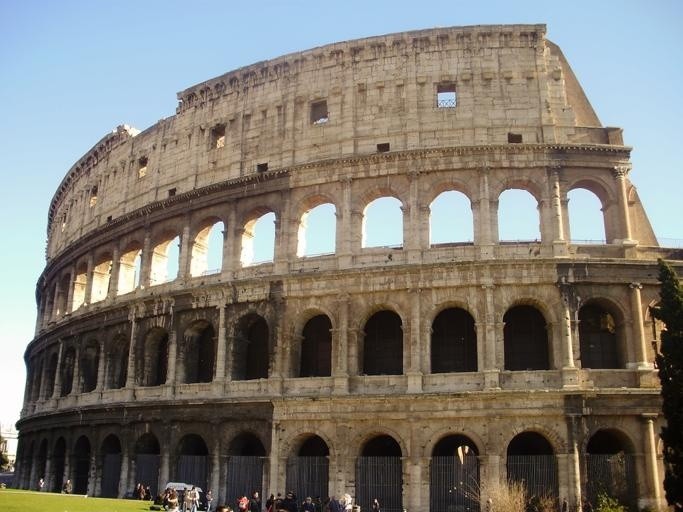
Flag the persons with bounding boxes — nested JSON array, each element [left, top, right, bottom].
[[582, 497, 594, 512], [133, 482, 380, 512], [36, 478, 44, 490], [562, 498, 569, 512], [63, 479, 72, 494]]
